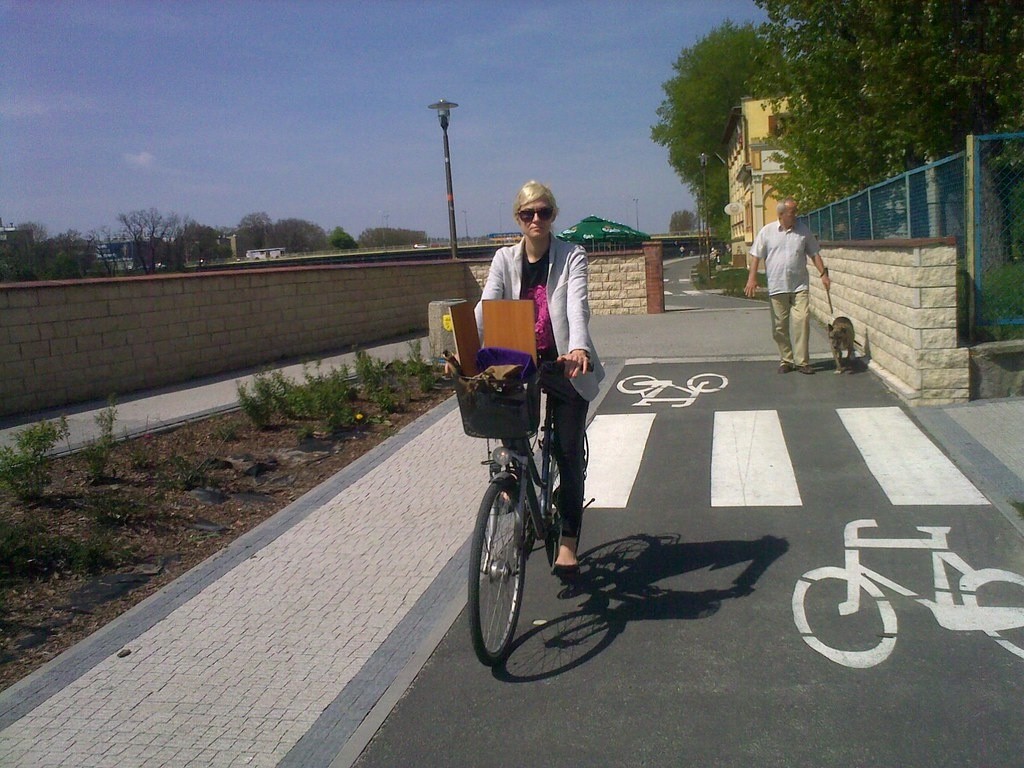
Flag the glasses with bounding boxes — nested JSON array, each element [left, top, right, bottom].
[[518, 207, 553, 222]]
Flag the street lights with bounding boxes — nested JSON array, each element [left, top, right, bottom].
[[697, 153, 711, 265], [427, 99, 459, 258]]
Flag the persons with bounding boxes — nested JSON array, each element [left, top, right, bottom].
[[679, 246, 685, 258], [443, 179, 607, 586], [743, 198, 831, 376]]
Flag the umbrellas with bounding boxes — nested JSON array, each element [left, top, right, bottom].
[[556, 213, 651, 245]]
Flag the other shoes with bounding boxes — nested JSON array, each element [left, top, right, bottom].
[[554, 556, 579, 571], [499, 496, 514, 512]]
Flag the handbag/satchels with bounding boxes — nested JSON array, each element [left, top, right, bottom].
[[458, 365, 519, 391]]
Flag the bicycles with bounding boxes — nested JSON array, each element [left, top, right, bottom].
[[455, 357, 596, 665]]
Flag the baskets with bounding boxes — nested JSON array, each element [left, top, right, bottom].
[[452, 373, 541, 440]]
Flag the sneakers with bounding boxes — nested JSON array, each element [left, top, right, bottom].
[[778, 366, 792, 373], [795, 365, 815, 373]]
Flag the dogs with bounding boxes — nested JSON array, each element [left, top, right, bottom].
[[827, 316, 855, 374]]
